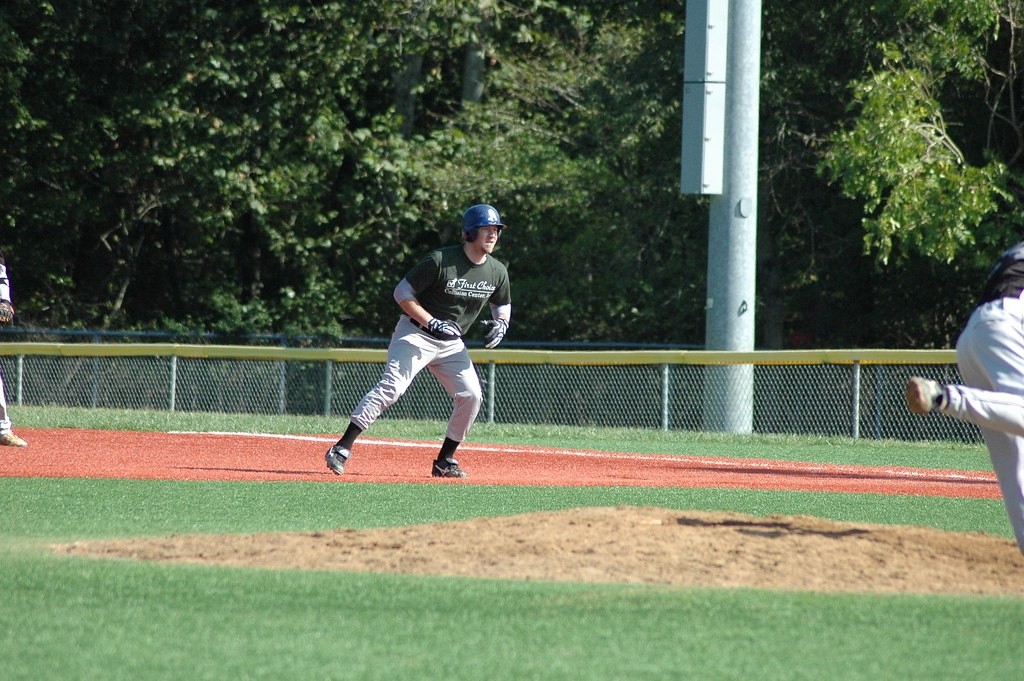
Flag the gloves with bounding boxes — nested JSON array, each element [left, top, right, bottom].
[[479, 317, 509, 348], [428, 319, 463, 339], [0, 299, 14, 322]]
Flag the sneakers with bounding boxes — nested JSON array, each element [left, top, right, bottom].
[[431, 458, 466, 477], [325, 446, 350, 475], [907, 375, 943, 414], [0, 432, 27, 447]]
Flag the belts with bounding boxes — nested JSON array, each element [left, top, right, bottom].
[[410, 317, 446, 341]]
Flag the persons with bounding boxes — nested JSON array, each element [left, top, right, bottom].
[[906, 241, 1024, 557], [0, 265, 28, 448], [324, 204, 511, 478]]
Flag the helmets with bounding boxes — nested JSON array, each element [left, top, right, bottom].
[[461, 204, 507, 242]]
